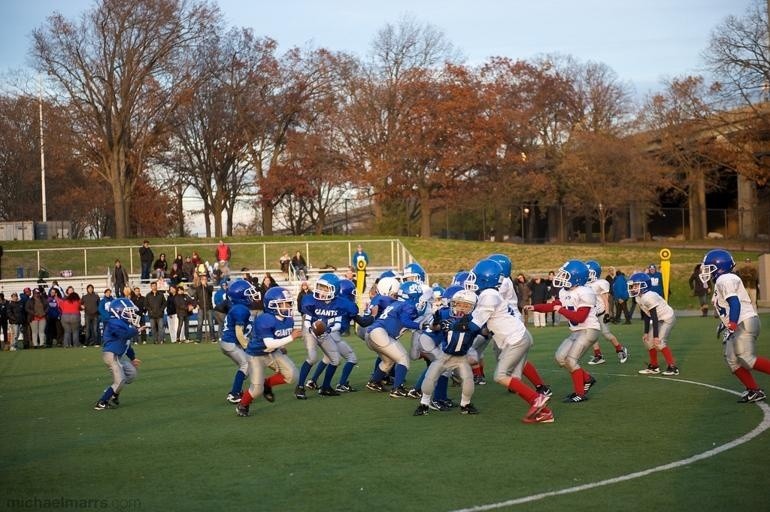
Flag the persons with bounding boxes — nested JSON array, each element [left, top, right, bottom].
[[191, 250, 201, 267], [413, 289, 489, 416], [626, 273, 680, 376], [146, 282, 167, 344], [406, 287, 445, 399], [644, 264, 664, 299], [297, 282, 314, 330], [182, 256, 195, 281], [48, 281, 66, 298], [92, 298, 147, 411], [353, 245, 369, 273], [344, 270, 359, 336], [80, 284, 101, 349], [25, 288, 49, 349], [452, 272, 486, 385], [174, 255, 183, 270], [214, 282, 229, 306], [261, 276, 279, 291], [613, 270, 631, 326], [544, 272, 560, 326], [403, 263, 458, 367], [584, 260, 628, 365], [20, 288, 34, 349], [213, 262, 222, 276], [513, 274, 532, 327], [442, 258, 554, 423], [45, 288, 63, 348], [235, 287, 298, 417], [248, 276, 265, 318], [364, 281, 430, 398], [698, 250, 770, 404], [241, 267, 252, 283], [131, 287, 148, 346], [204, 260, 213, 275], [524, 260, 601, 404], [221, 280, 261, 405], [54, 292, 81, 349], [293, 273, 341, 400], [358, 277, 405, 386], [365, 286, 379, 318], [166, 285, 186, 344], [37, 267, 49, 289], [98, 289, 115, 333], [531, 276, 549, 328], [689, 264, 712, 317], [7, 292, 24, 352], [305, 279, 357, 392], [154, 253, 168, 279], [291, 251, 309, 280], [0, 293, 9, 344], [606, 266, 619, 323], [174, 286, 194, 345], [213, 300, 232, 341], [279, 251, 292, 281], [139, 240, 154, 284], [194, 275, 218, 344], [410, 286, 464, 412], [739, 258, 758, 315], [111, 259, 129, 298], [170, 263, 183, 283], [219, 259, 231, 280], [489, 254, 553, 397], [215, 240, 232, 267], [195, 263, 201, 275]]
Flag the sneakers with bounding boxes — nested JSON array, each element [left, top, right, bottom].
[[263, 378, 274, 402], [305, 380, 318, 389], [588, 355, 605, 365], [431, 402, 445, 411], [736, 389, 767, 403], [616, 347, 628, 364], [441, 399, 453, 407], [366, 381, 384, 392], [335, 383, 355, 392], [475, 376, 487, 385], [661, 367, 679, 376], [112, 395, 119, 404], [638, 367, 661, 375], [461, 405, 481, 415], [235, 403, 249, 416], [526, 394, 551, 419], [295, 385, 305, 399], [94, 401, 109, 410], [408, 389, 422, 399], [318, 386, 339, 396], [562, 394, 586, 404], [583, 378, 596, 393], [413, 406, 429, 417], [521, 409, 554, 424], [225, 393, 243, 404], [390, 388, 408, 398], [533, 387, 552, 397]]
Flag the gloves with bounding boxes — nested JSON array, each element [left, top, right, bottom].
[[310, 329, 327, 342], [721, 328, 735, 344], [716, 323, 727, 339], [432, 311, 441, 327]]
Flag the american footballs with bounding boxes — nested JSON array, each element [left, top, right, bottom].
[[311, 320, 326, 336]]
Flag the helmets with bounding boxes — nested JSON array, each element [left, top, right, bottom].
[[403, 264, 425, 284], [449, 291, 477, 317], [312, 273, 340, 300], [698, 250, 735, 284], [584, 261, 601, 282], [380, 271, 404, 285], [376, 277, 401, 298], [398, 281, 423, 302], [227, 280, 261, 304], [108, 298, 142, 327], [338, 279, 356, 299], [451, 272, 469, 286], [626, 273, 651, 297], [553, 260, 589, 288], [488, 255, 512, 278], [441, 286, 466, 307], [263, 286, 294, 317], [431, 287, 446, 305], [464, 259, 504, 292]]
